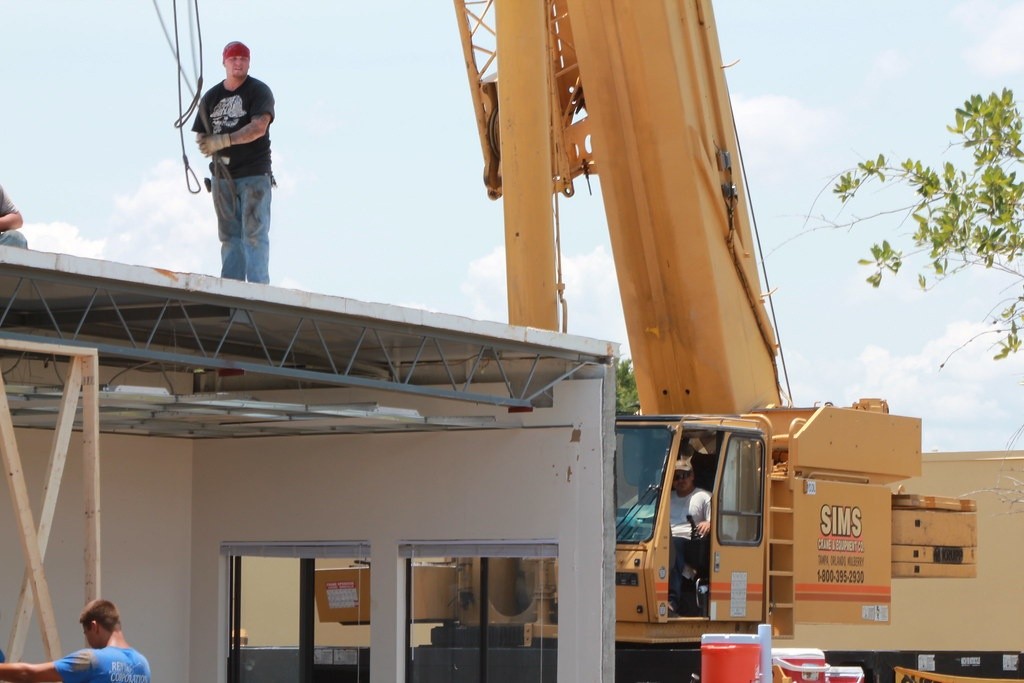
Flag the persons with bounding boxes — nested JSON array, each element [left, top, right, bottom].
[[0, 182, 30, 249], [0, 596, 152, 683], [647, 457, 714, 616], [190, 41, 275, 286]]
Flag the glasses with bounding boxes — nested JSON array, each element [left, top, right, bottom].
[[675, 471, 691, 479]]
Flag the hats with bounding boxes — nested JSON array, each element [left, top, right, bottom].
[[223, 43, 250, 62], [675, 459, 692, 471]]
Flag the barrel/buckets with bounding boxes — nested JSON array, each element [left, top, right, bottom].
[[700, 633, 762, 683], [772, 648, 831, 683], [825, 666, 865, 683]]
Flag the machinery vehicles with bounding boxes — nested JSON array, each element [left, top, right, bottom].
[[313, 0, 1023, 683]]
